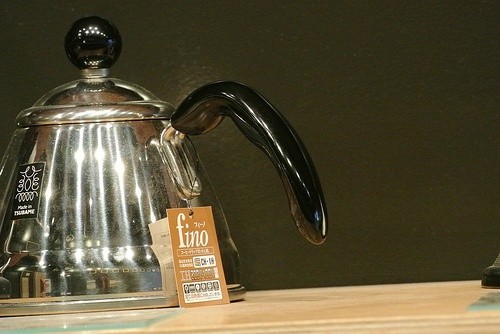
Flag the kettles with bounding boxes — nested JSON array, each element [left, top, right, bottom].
[[0, 15, 329, 317]]
